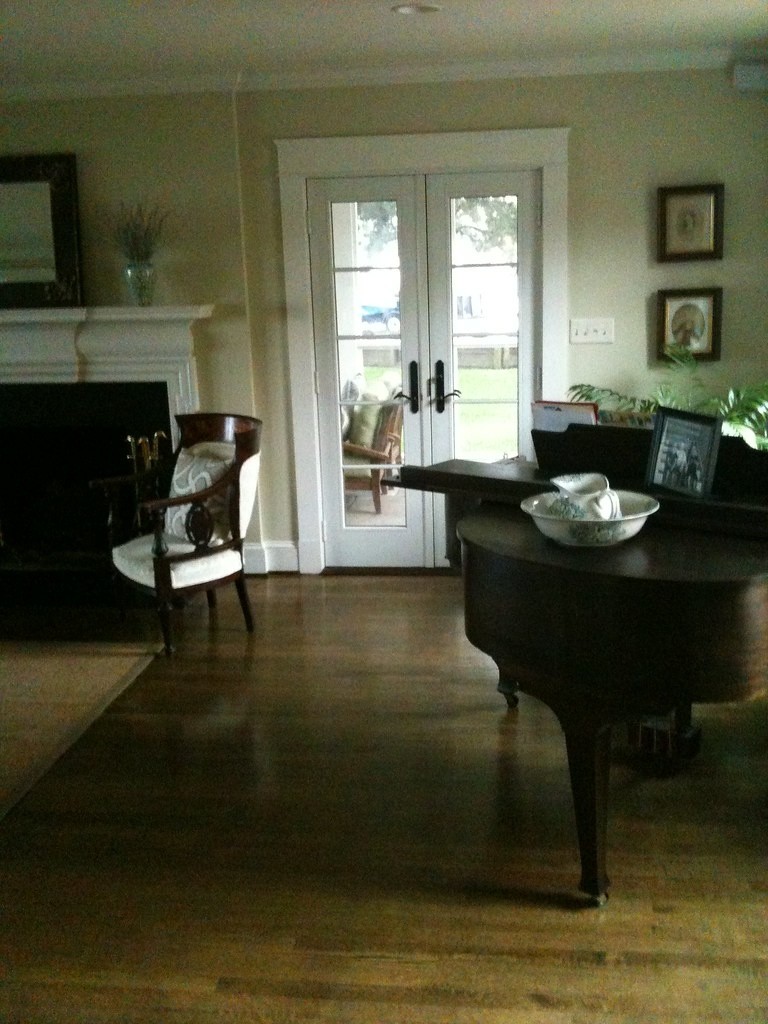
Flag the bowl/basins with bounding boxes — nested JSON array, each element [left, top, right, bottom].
[[519, 489, 661, 546]]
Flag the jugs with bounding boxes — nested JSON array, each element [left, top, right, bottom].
[[551, 472, 620, 520]]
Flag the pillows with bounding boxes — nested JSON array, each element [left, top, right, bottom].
[[349, 394, 382, 449], [164, 447, 234, 547]]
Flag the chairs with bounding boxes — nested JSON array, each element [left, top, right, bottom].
[[89, 413, 262, 657], [342, 404, 403, 513]]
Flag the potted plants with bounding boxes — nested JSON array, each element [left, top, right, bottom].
[[106, 200, 174, 306]]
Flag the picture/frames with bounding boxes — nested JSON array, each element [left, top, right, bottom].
[[657, 287, 723, 361], [645, 405, 722, 497], [658, 184, 724, 262]]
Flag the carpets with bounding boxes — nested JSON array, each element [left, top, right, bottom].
[[0, 638, 164, 822]]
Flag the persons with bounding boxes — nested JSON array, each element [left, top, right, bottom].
[[664, 440, 701, 492]]
